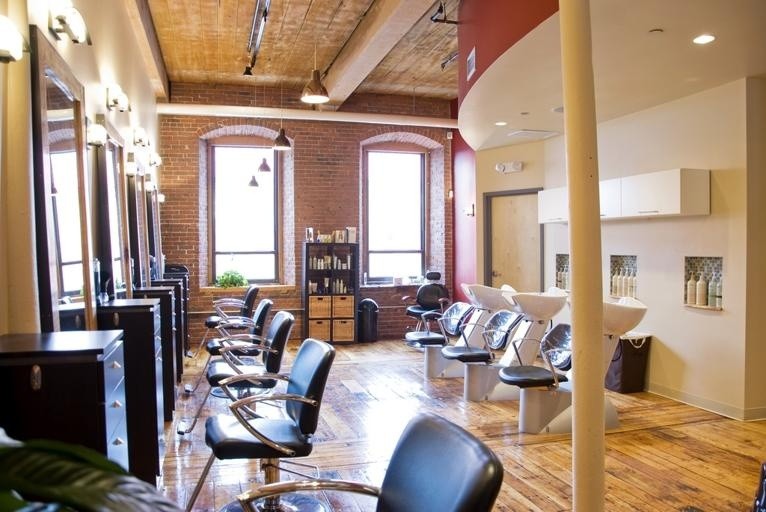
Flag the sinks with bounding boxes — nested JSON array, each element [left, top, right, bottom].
[[565, 295, 649, 339], [460, 282, 518, 310], [501, 287, 569, 323]]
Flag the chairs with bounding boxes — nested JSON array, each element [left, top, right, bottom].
[[184, 297, 274, 393], [498, 323, 572, 389], [400, 271, 452, 342], [176, 310, 294, 435], [440, 310, 525, 363], [235, 410, 504, 512], [184, 337, 336, 512], [187, 283, 260, 358], [404, 301, 474, 348]]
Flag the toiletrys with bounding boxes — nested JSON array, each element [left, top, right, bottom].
[[557, 265, 570, 291], [695, 270, 708, 306], [707, 272, 717, 307], [611, 266, 637, 299], [308, 254, 344, 294], [716, 271, 723, 307], [308, 252, 353, 294], [686, 271, 696, 305]]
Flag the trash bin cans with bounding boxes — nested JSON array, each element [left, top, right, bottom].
[[358, 298, 379, 343]]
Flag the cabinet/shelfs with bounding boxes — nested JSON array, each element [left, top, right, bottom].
[[621, 168, 711, 221], [97, 298, 167, 489], [151, 278, 184, 386], [301, 241, 359, 345], [537, 187, 568, 224], [1, 328, 131, 476], [163, 273, 190, 357], [600, 176, 621, 222], [134, 286, 177, 423]]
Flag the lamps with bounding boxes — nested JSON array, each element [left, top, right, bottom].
[[47, 0, 93, 46], [258, 54, 271, 173], [0, 15, 29, 64], [134, 127, 149, 148], [247, 67, 258, 189], [242, 65, 252, 76], [273, 32, 291, 152], [106, 84, 130, 113], [495, 160, 522, 174], [299, 0, 329, 105]]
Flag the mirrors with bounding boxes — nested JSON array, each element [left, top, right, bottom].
[[128, 152, 152, 289], [145, 173, 163, 279], [96, 113, 133, 300], [30, 23, 97, 333]]
